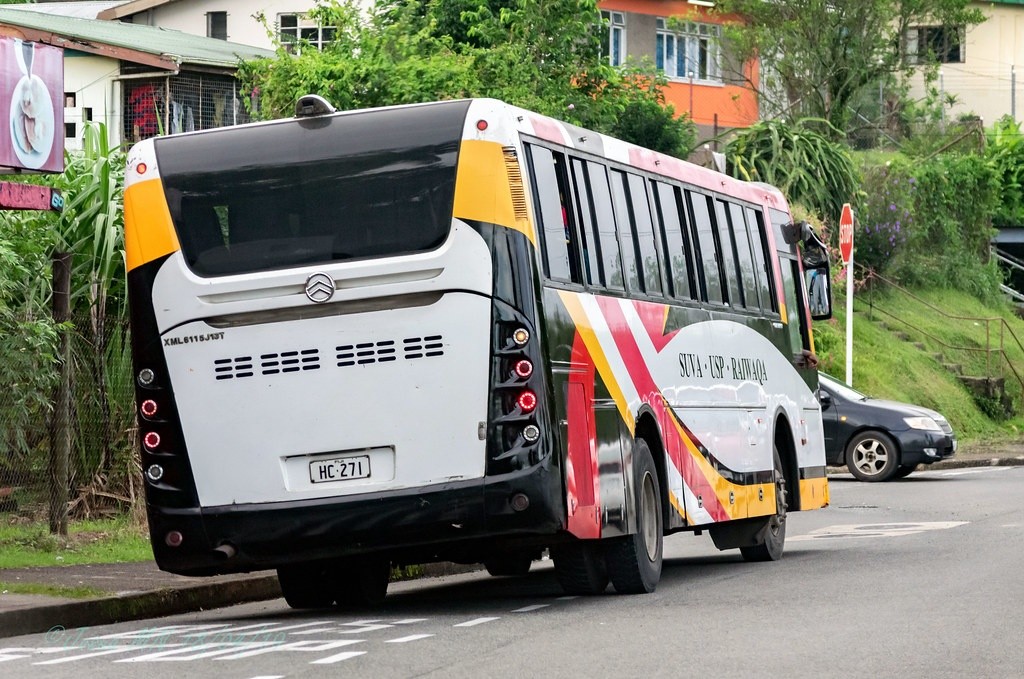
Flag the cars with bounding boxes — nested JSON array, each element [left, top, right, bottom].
[[812, 368, 958, 482]]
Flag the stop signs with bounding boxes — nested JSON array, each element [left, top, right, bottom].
[[838, 202, 856, 267]]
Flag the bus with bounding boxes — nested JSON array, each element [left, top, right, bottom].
[[116, 95, 830, 613]]
